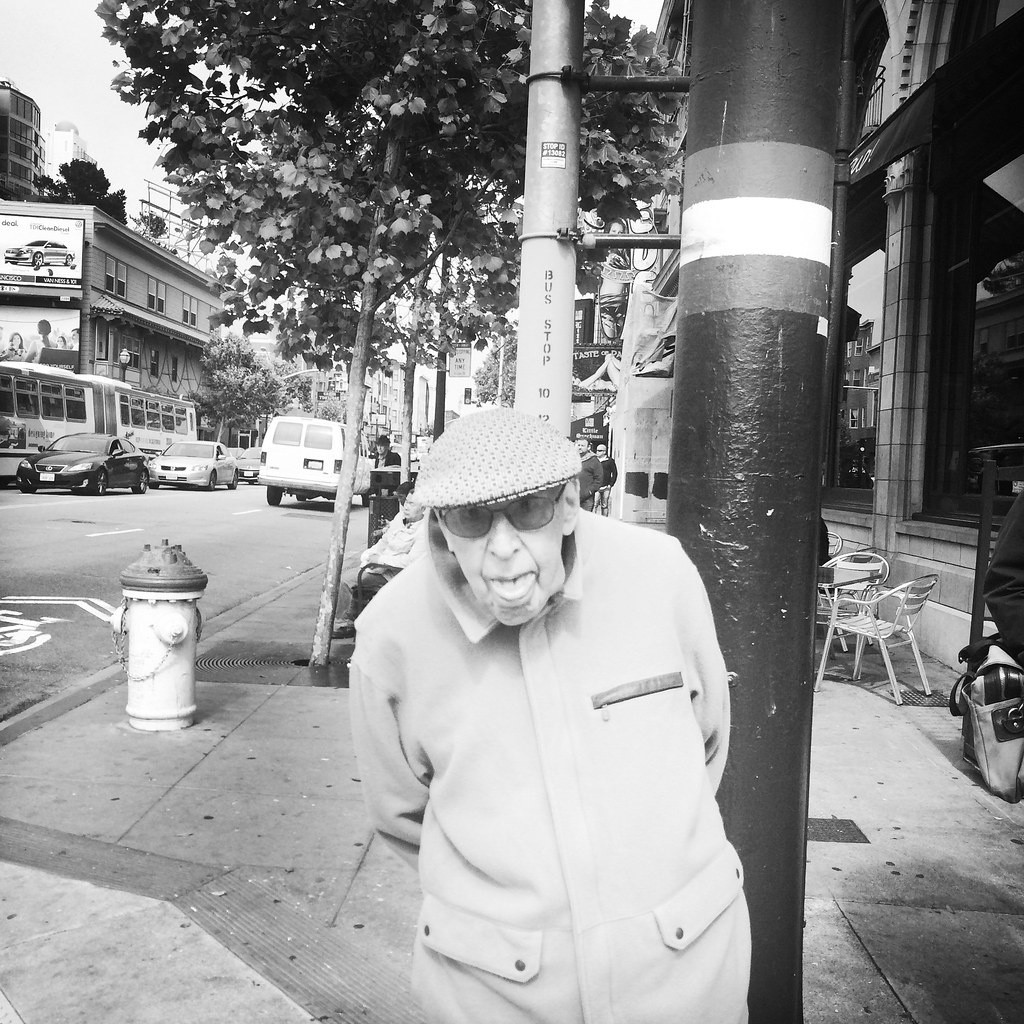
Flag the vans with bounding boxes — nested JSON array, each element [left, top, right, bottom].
[[257, 416, 375, 506]]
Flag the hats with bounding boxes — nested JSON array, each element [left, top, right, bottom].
[[413, 407, 583, 509], [374, 435, 391, 445]]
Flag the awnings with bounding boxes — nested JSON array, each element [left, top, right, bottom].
[[848, 4, 1024, 184]]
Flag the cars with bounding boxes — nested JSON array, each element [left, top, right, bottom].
[[5, 240, 75, 268], [15, 433, 151, 496], [390, 443, 419, 463], [146, 441, 239, 491], [227, 447, 262, 485]]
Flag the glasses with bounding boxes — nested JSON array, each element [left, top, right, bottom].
[[598, 450, 605, 452], [434, 482, 573, 540]]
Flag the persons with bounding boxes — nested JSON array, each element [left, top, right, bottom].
[[374, 434, 401, 469], [349, 410, 752, 1022], [331, 481, 425, 639], [0, 319, 80, 364], [577, 436, 603, 512], [591, 444, 617, 518], [982, 487, 1024, 668]]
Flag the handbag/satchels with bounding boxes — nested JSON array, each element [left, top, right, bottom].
[[949, 639, 1024, 804]]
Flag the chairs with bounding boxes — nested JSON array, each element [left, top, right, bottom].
[[813, 532, 940, 707]]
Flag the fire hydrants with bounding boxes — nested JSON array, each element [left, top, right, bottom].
[[109, 539, 210, 732]]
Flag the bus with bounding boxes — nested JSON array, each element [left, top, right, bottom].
[[0, 361, 198, 490]]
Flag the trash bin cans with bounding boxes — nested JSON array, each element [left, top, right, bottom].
[[367, 470, 418, 550]]
